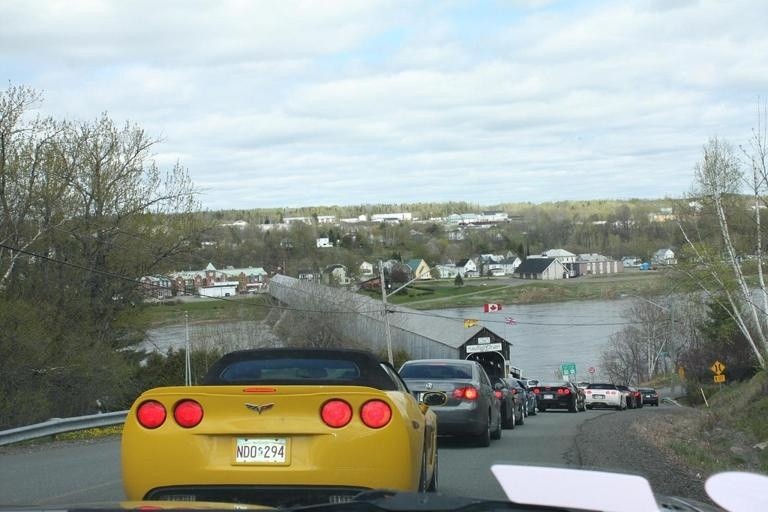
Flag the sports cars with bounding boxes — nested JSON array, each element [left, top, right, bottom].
[[120, 345, 448, 504]]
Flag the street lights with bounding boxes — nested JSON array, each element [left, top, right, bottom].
[[621, 293, 675, 397], [376, 258, 455, 366]]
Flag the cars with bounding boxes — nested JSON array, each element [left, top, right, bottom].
[[577, 382, 659, 410], [487, 377, 538, 427], [532, 382, 581, 412], [397, 359, 502, 447]]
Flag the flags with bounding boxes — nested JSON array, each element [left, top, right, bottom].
[[464, 319, 481, 329], [484, 303, 501, 313], [505, 317, 519, 328]]
